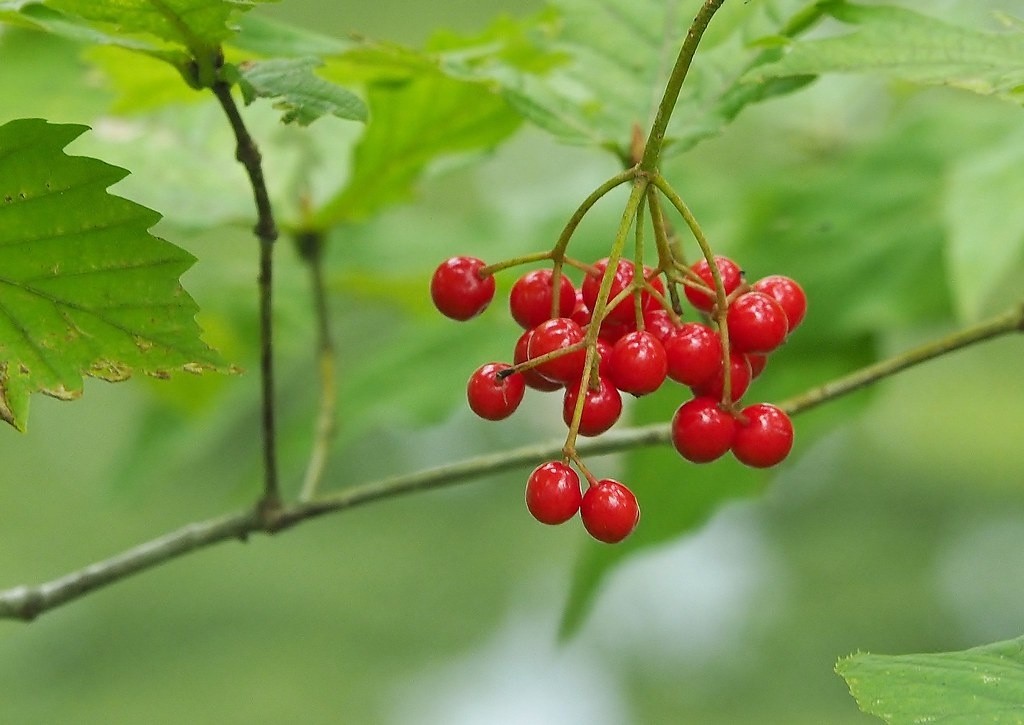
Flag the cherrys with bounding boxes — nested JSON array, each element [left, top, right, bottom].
[[432, 255, 805, 543]]
